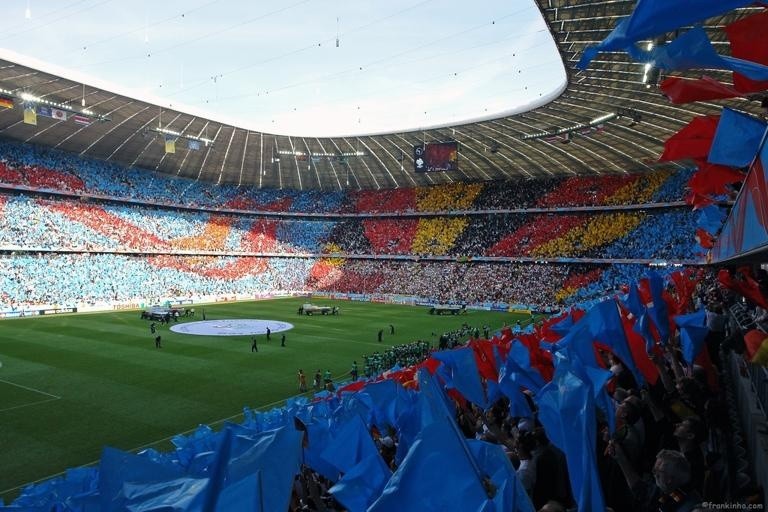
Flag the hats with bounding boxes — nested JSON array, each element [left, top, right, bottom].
[[625, 394, 644, 413]]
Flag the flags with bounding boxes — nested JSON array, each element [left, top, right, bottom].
[[577, 1, 767, 166]]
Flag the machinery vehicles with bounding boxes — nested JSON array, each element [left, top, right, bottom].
[[430, 301, 463, 316]]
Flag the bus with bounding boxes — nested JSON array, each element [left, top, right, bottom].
[[303, 303, 331, 316]]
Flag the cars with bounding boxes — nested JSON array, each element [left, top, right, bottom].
[[142, 306, 184, 324]]
